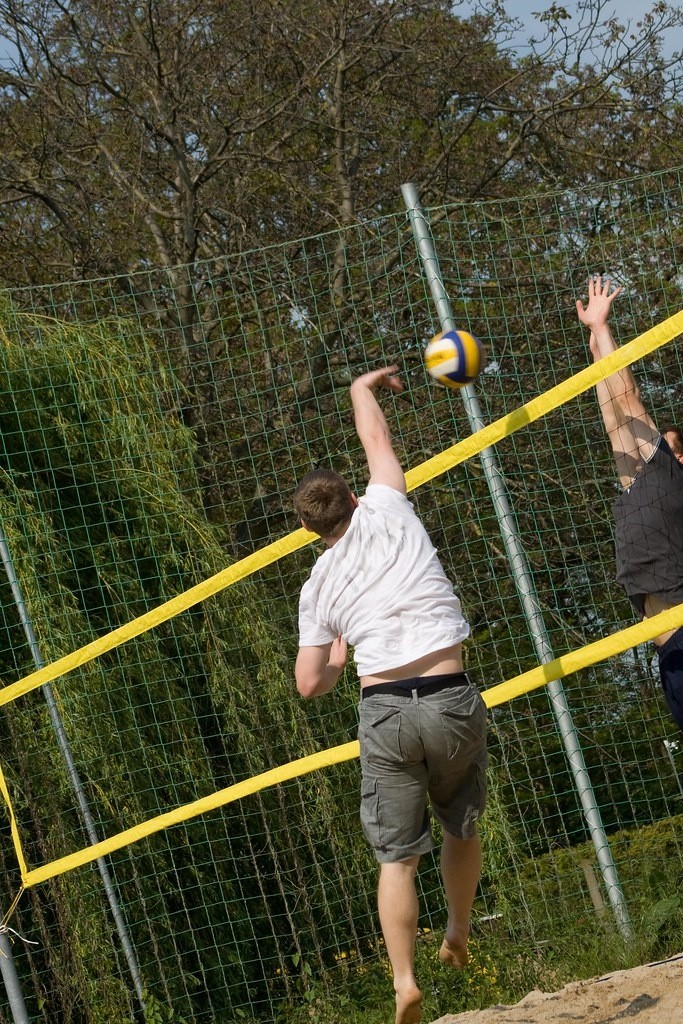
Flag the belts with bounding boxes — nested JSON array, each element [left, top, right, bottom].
[[362, 673, 468, 698]]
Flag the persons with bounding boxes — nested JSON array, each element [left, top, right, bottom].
[[292, 361, 488, 1024], [574, 275, 683, 735]]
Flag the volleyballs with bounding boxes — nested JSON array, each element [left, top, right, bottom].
[[423, 329, 487, 389]]
